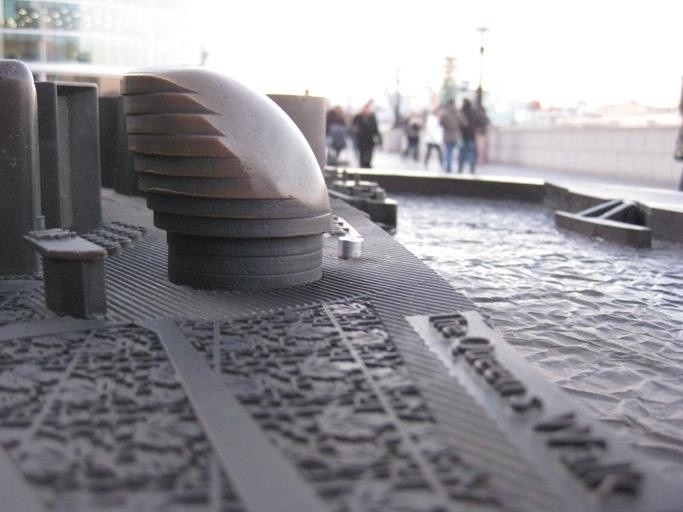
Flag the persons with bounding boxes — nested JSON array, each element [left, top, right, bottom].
[[674, 78, 683, 192], [325, 98, 492, 176]]
